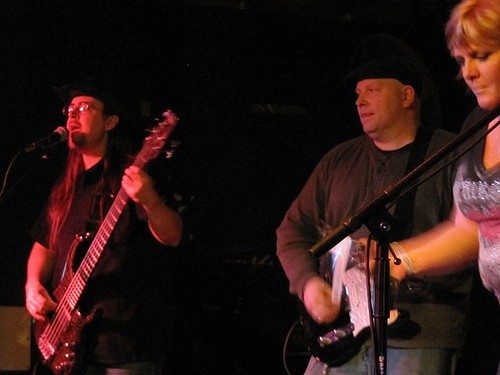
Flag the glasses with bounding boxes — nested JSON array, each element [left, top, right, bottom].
[[60, 101, 105, 116]]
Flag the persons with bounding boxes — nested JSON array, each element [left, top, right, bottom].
[[274, 52, 476, 375], [25, 83, 194, 375], [352, 0, 500, 375]]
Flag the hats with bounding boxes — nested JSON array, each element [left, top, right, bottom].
[[345, 56, 417, 87]]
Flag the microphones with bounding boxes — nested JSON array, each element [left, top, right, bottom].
[[17, 126, 69, 157]]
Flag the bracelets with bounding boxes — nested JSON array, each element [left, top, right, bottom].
[[391, 241, 416, 278]]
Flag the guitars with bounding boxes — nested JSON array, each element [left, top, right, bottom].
[[33, 110, 179, 375]]
